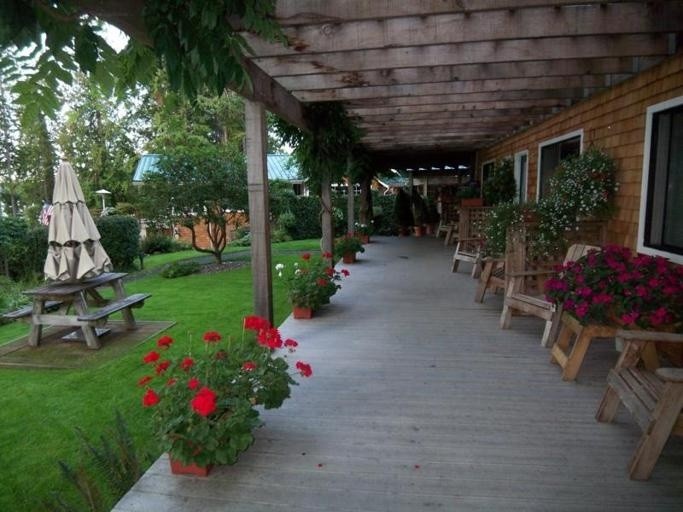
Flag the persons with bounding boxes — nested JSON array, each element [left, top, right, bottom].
[[173, 222, 180, 240]]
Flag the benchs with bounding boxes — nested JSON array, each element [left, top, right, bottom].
[[77, 293, 151, 322], [3, 299, 61, 321]]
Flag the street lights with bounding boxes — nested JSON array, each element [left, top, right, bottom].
[[95, 188, 112, 212]]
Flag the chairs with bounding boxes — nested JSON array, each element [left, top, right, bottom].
[[436, 219, 458, 246]]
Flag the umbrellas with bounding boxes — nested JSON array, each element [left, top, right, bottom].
[[42, 157, 113, 285]]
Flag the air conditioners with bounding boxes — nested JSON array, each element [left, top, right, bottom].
[[413, 211, 423, 237]]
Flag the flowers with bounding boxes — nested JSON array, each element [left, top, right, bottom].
[[136, 313, 313, 469], [275, 250, 350, 311], [353, 219, 375, 235], [333, 231, 367, 259], [473, 144, 620, 260]]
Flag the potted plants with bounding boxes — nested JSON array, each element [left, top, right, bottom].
[[456, 184, 484, 207], [422, 205, 441, 235]]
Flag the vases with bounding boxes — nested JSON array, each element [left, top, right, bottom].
[[343, 250, 355, 263], [168, 436, 211, 477], [292, 305, 312, 319], [358, 235, 369, 244]]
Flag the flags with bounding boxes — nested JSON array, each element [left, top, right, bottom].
[[36, 202, 53, 226]]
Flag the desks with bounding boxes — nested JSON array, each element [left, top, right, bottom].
[[22, 272, 152, 350]]
